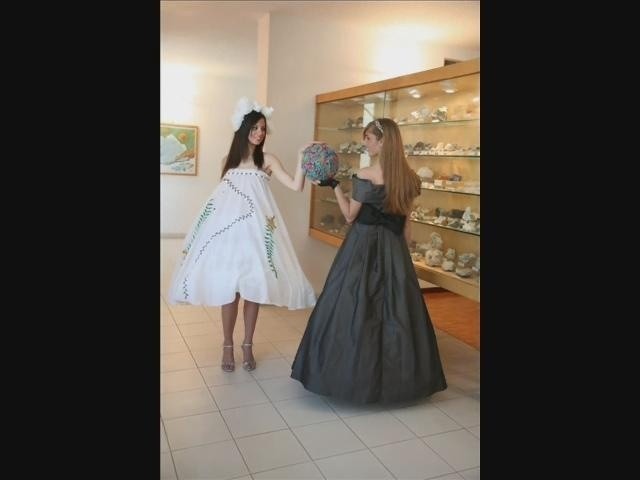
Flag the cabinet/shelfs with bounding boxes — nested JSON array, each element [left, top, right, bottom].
[[307, 58, 481, 309]]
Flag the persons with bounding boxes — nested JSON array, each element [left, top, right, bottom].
[[169, 100, 318, 373], [290, 119, 448, 403]]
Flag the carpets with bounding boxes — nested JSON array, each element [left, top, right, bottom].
[[421, 291, 481, 354]]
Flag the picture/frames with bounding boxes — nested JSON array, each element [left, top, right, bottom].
[[160, 123, 200, 178]]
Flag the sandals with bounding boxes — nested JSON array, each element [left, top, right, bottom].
[[221, 343, 235, 372], [242, 343, 256, 371]]
